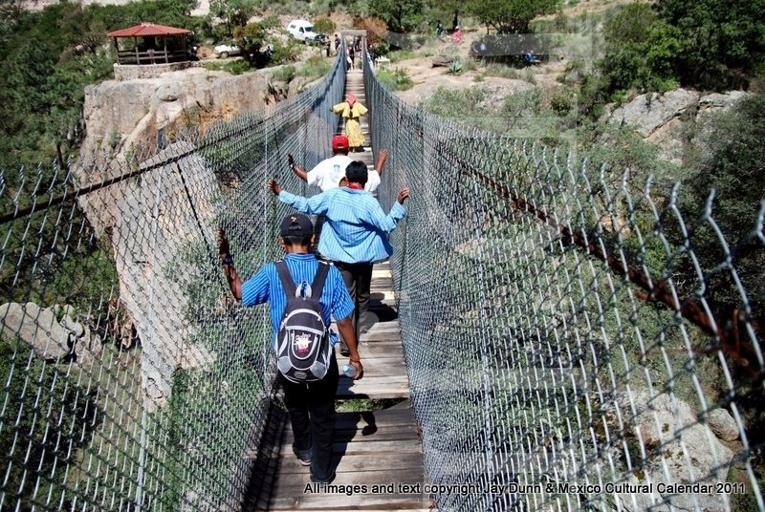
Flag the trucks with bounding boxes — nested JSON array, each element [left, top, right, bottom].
[[288, 20, 322, 46]]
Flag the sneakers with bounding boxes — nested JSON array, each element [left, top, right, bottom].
[[297, 457, 312, 466], [339, 341, 350, 355]]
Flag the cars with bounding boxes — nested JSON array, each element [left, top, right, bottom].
[[213, 40, 243, 59], [470, 34, 549, 68]]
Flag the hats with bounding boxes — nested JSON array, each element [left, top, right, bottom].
[[279, 214, 313, 237], [332, 136, 349, 150]]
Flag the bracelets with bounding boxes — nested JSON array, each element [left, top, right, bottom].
[[349, 358, 361, 364], [221, 256, 234, 265]]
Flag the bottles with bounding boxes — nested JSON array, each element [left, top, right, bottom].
[[342, 365, 357, 377]]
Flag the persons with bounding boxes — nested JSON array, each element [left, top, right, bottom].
[[321, 35, 361, 71], [216, 212, 361, 490], [287, 137, 388, 194], [260, 160, 411, 357], [331, 91, 369, 152], [452, 26, 463, 44]]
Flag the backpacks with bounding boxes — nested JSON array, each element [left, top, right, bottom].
[[274, 260, 340, 405]]
